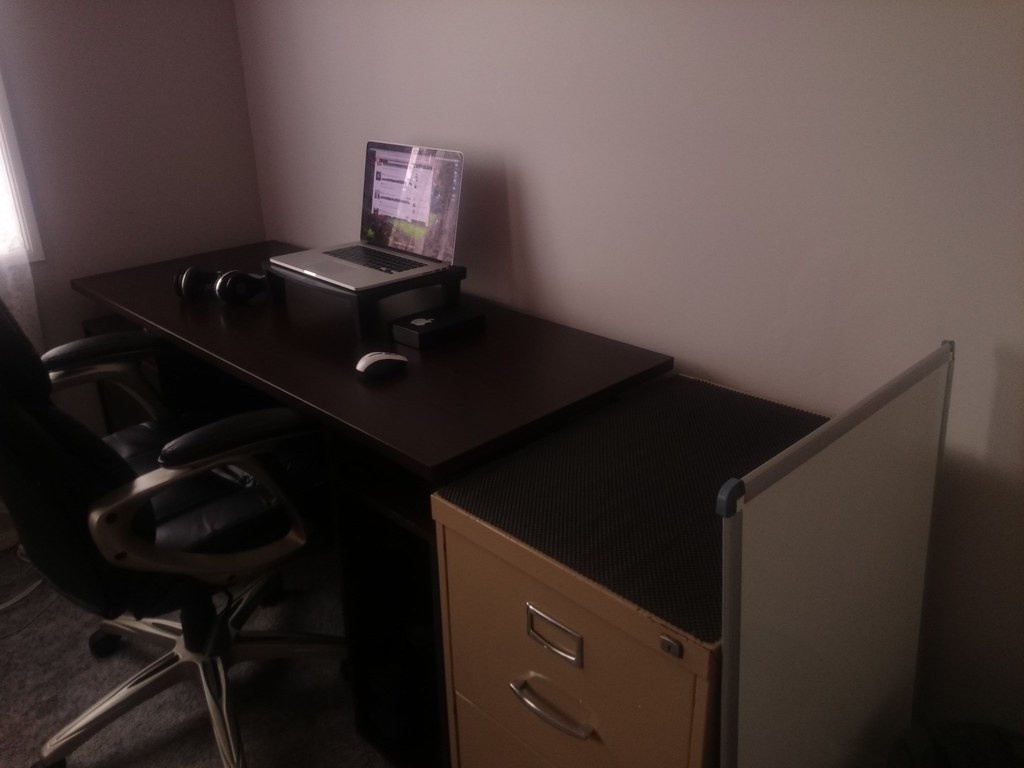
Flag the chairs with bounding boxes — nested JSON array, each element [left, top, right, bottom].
[[0, 302, 347, 768]]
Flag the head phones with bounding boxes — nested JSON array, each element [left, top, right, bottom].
[[174, 267, 278, 306]]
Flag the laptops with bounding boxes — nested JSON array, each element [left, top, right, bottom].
[[267, 140, 467, 292]]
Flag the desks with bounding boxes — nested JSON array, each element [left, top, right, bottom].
[[70, 240, 675, 768]]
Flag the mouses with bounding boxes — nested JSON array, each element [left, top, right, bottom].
[[352, 349, 408, 381]]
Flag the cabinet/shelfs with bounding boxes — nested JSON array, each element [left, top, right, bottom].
[[430, 367, 833, 768]]
